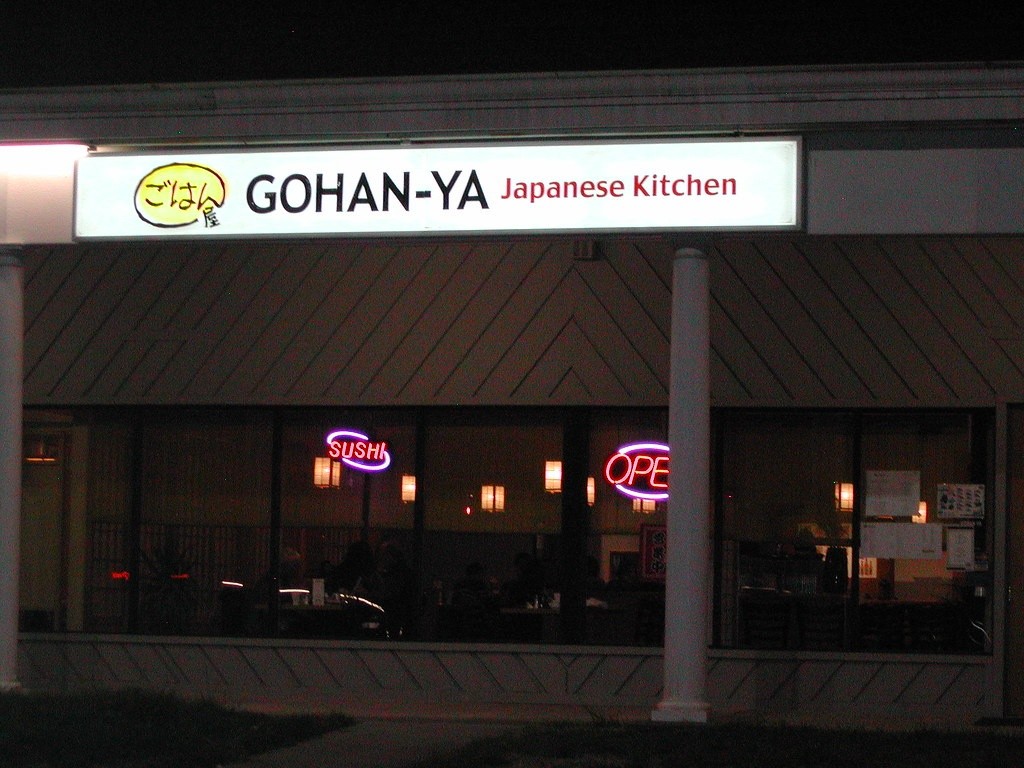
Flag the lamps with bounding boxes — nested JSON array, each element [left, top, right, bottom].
[[314, 456, 657, 515], [834, 482, 853, 512]]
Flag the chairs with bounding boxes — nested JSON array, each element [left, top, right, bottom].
[[634, 595, 959, 652]]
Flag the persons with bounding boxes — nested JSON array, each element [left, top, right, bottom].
[[343, 540, 647, 642]]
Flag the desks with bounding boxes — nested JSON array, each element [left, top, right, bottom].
[[499, 608, 556, 615], [286, 603, 344, 639]]
